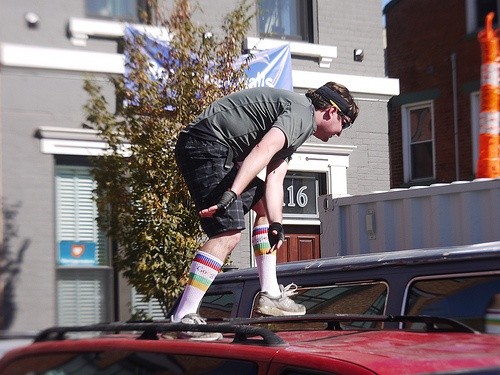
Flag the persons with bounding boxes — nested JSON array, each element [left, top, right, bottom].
[[158, 81, 359, 341]]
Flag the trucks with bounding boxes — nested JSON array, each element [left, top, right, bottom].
[[318, 177, 500, 259]]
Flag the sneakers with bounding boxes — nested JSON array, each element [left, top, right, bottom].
[[254, 283, 306, 316], [157, 311, 222, 342]]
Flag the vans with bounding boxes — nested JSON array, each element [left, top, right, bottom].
[[167, 239, 500, 333]]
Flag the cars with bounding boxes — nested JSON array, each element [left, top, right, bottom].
[[0, 313, 498, 375]]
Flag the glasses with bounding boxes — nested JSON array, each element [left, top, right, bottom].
[[331, 101, 351, 129]]
